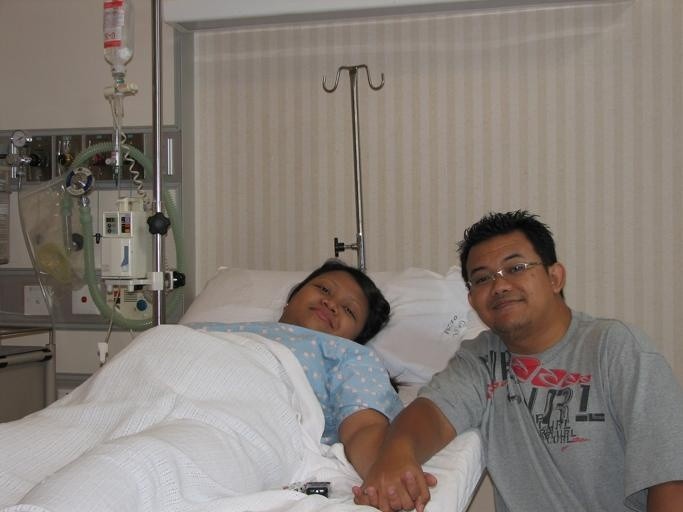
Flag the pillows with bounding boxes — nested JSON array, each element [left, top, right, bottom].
[[177, 268, 473, 382]]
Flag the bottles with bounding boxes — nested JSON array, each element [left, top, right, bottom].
[[103, 1, 134, 76]]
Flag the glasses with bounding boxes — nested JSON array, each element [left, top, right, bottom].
[[465, 262, 541, 289]]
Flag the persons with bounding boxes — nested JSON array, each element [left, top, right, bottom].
[[174, 257, 438, 512], [353, 210, 683, 512]]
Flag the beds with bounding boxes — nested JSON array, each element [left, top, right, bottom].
[[0, 267, 488, 512]]
